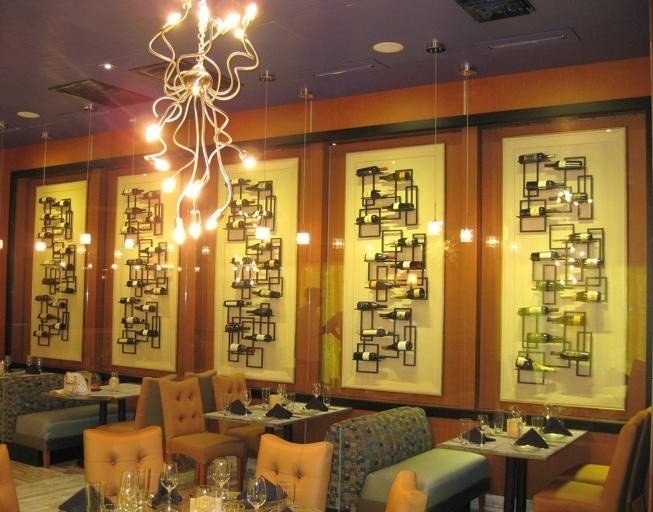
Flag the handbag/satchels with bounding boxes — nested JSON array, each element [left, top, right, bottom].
[[63, 372, 88, 395]]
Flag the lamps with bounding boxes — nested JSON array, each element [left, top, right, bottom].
[[458, 60, 476, 243], [427, 40, 444, 236], [257, 72, 275, 242], [98, 62, 116, 73], [124, 114, 140, 249], [143, 0, 258, 245], [33, 96, 52, 255], [77, 99, 94, 245], [297, 83, 315, 246]]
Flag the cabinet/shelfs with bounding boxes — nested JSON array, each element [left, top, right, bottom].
[[222, 178, 282, 369], [118, 187, 168, 354], [356, 163, 428, 372], [34, 195, 77, 346]]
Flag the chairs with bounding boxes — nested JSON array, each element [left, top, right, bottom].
[[0, 370, 653, 510]]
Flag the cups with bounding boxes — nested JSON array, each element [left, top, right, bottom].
[[456, 404, 567, 451], [23, 355, 43, 374], [223, 383, 332, 415]]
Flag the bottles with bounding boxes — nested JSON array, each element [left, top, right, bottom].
[[3, 356, 13, 373], [224, 178, 280, 354], [33, 197, 75, 337], [352, 167, 426, 361], [116, 188, 166, 344], [515, 152, 602, 372], [91, 370, 120, 393]]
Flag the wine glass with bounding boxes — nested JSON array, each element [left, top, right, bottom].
[[84, 457, 296, 512]]
